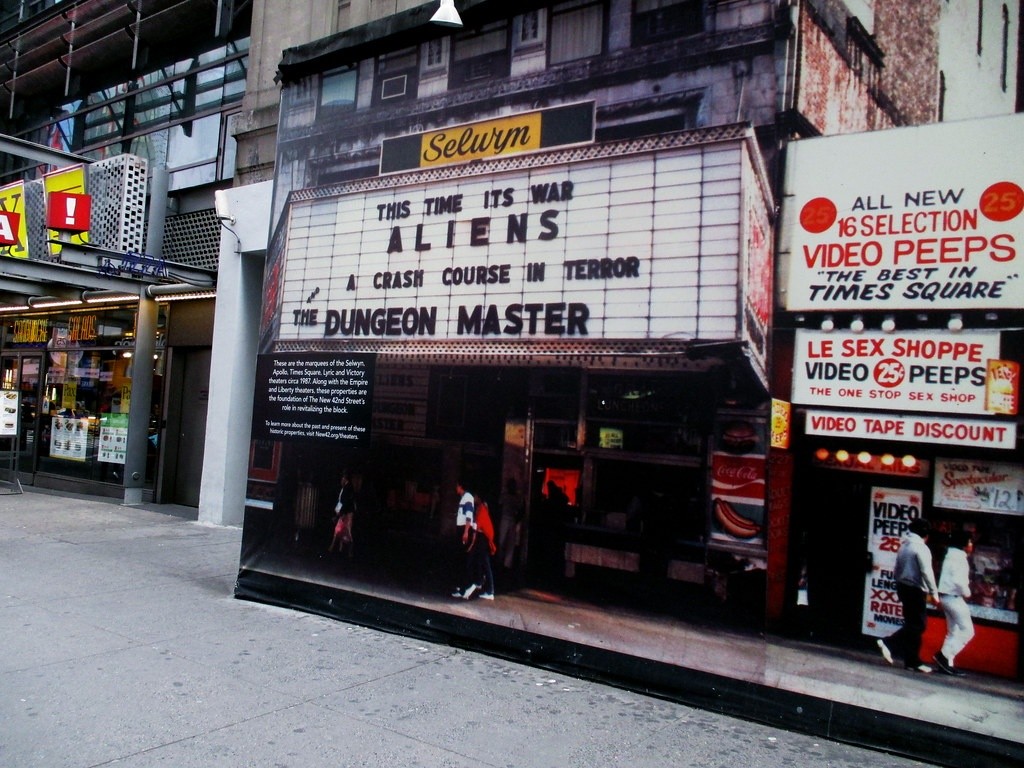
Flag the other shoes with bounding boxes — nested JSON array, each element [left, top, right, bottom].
[[463, 584, 477, 600], [877, 639, 894, 664], [937, 666, 962, 676], [933, 651, 953, 674], [479, 593, 494, 600], [451, 591, 462, 596], [906, 662, 933, 673]]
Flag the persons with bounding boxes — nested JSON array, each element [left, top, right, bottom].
[[497, 478, 526, 581], [429, 471, 465, 570], [449, 481, 496, 601], [329, 473, 357, 552], [932, 536, 975, 676], [877, 519, 940, 673]]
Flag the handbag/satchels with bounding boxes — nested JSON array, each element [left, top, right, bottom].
[[515, 511, 524, 523], [334, 488, 344, 514]]
